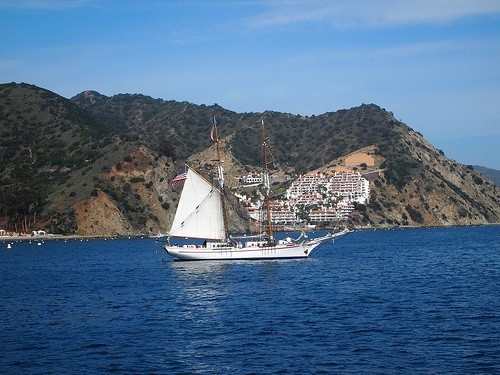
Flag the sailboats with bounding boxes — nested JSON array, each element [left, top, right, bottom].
[[164, 113, 356, 260]]
